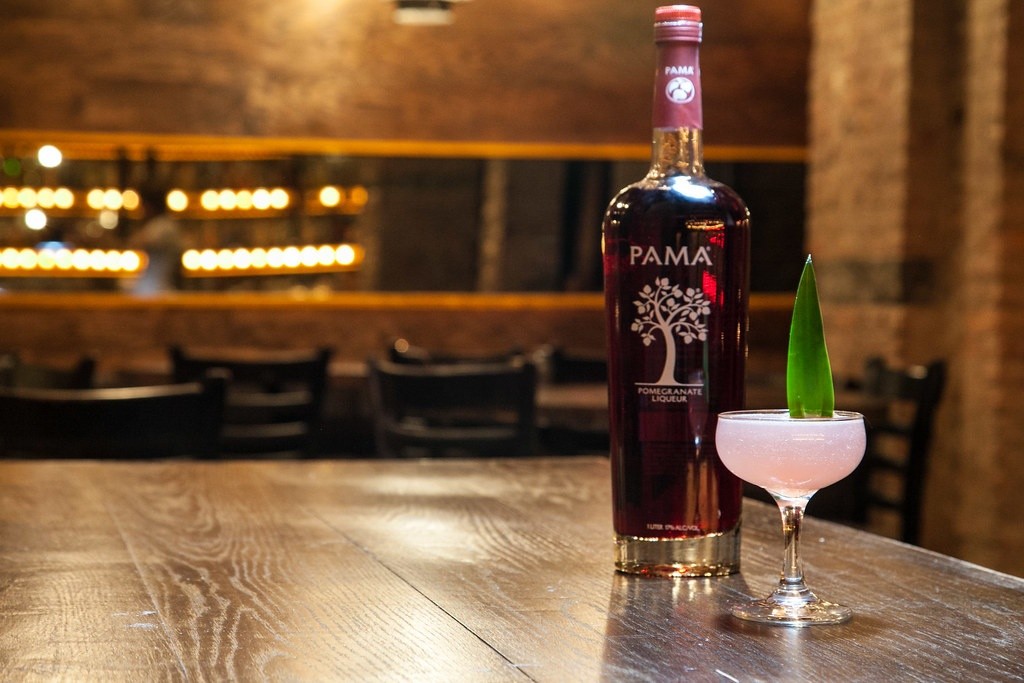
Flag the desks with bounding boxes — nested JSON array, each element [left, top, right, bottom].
[[0, 460, 1024, 683]]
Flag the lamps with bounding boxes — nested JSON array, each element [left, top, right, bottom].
[[394, 0, 451, 27]]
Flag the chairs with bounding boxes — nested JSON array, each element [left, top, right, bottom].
[[167, 338, 339, 466], [833, 355, 947, 545], [369, 337, 534, 456], [0, 382, 201, 466]]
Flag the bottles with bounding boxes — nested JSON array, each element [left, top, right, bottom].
[[602, 6, 749, 576]]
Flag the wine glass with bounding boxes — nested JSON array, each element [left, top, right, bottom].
[[717, 411, 865, 625]]
